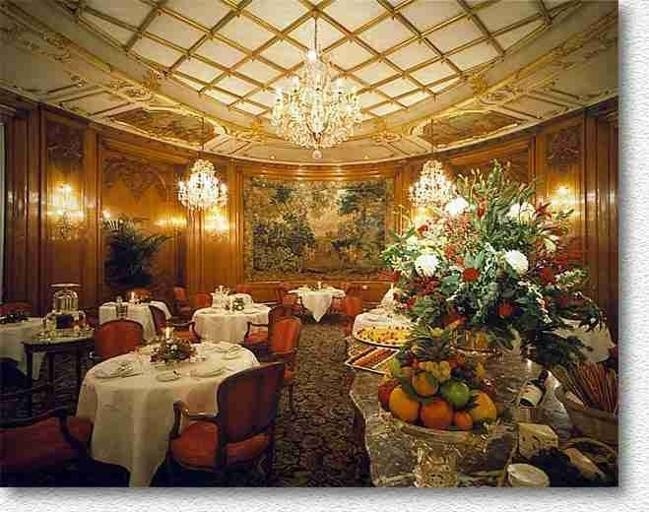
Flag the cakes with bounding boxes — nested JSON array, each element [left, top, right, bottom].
[[518, 422, 559, 459]]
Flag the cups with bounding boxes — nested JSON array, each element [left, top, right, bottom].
[[507, 463, 549, 486]]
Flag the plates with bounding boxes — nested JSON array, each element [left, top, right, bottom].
[[94, 343, 251, 382], [200, 303, 266, 315]]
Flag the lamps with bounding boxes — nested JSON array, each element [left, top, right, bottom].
[[270, 9, 361, 160], [407, 119, 457, 212], [51, 175, 80, 245], [178, 117, 228, 211]]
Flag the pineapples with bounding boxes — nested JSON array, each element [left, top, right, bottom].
[[413, 330, 452, 383]]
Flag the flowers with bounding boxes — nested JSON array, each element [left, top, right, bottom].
[[377, 157, 608, 369]]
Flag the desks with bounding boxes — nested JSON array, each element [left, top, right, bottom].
[[75, 340, 259, 486], [3, 297, 97, 419], [344, 335, 618, 488], [83, 292, 173, 339], [193, 302, 271, 345]]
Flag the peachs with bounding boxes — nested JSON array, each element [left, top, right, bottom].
[[454, 410, 473, 429]]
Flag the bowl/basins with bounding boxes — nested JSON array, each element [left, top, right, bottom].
[[555, 380, 619, 444], [559, 436, 618, 486]]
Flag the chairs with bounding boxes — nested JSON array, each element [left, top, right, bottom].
[[274, 281, 365, 323], [148, 304, 194, 342], [171, 282, 211, 319], [2, 380, 93, 487], [246, 316, 302, 416], [88, 319, 143, 364], [244, 305, 298, 350], [169, 358, 286, 487]]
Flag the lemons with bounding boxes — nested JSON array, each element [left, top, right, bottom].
[[411, 372, 438, 395], [389, 385, 420, 422], [466, 389, 496, 425]]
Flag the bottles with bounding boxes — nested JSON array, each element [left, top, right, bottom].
[[518, 370, 548, 408]]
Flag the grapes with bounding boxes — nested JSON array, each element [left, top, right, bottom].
[[396, 351, 415, 367], [452, 361, 474, 383]]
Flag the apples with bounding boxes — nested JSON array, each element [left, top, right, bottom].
[[420, 396, 454, 429], [442, 380, 470, 408], [378, 380, 402, 412]]
